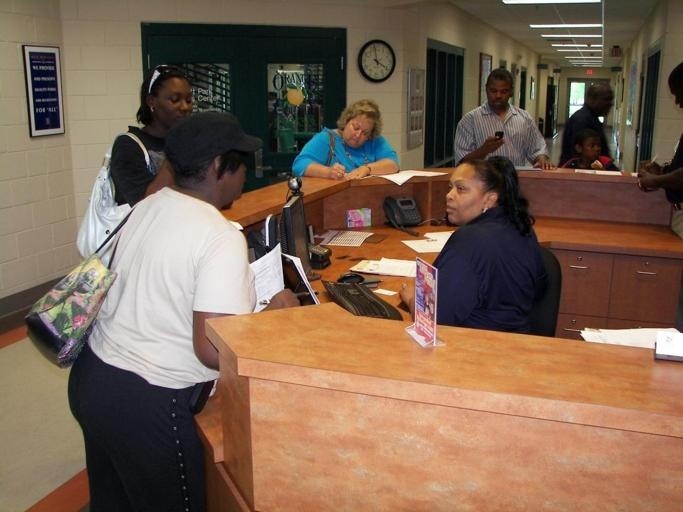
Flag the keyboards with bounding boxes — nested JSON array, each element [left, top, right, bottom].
[[321, 279, 404, 321]]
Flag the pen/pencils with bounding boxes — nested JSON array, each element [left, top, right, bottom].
[[359, 280, 384, 286], [330, 145, 341, 164], [651, 154, 659, 164], [259, 292, 312, 305]]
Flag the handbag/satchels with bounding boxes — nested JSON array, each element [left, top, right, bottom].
[[24, 203, 136, 368], [76, 132, 152, 258]]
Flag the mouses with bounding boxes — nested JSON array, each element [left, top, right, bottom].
[[337, 274, 365, 283]]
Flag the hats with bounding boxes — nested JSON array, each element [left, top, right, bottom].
[[166, 112, 261, 162]]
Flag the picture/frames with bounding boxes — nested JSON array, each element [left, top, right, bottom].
[[20, 44, 64, 137]]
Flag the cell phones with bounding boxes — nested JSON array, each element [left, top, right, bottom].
[[495, 132, 504, 140]]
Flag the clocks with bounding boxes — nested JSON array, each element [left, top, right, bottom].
[[357, 40, 396, 84]]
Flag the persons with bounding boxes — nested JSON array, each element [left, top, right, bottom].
[[636, 60, 683, 239], [560, 82, 616, 171], [559, 127, 620, 170], [289, 98, 402, 183], [109, 64, 194, 210], [398, 155, 551, 336], [67, 108, 302, 511], [453, 68, 559, 172]]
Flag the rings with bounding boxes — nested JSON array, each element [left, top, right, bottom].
[[401, 283, 408, 289], [638, 183, 641, 188], [637, 178, 640, 182], [337, 173, 341, 177]]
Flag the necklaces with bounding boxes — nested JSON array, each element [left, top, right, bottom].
[[342, 141, 369, 168]]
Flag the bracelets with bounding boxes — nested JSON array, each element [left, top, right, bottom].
[[362, 165, 372, 176]]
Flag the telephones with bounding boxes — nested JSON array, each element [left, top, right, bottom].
[[382, 195, 422, 226]]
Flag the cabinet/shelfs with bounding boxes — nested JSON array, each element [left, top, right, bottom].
[[550, 249, 683, 341]]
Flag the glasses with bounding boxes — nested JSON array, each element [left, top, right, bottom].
[[149, 65, 193, 95]]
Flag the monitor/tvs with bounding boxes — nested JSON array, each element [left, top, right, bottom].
[[280, 195, 313, 277]]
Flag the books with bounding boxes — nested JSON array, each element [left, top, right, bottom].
[[263, 246, 320, 306], [653, 331, 683, 362]]
